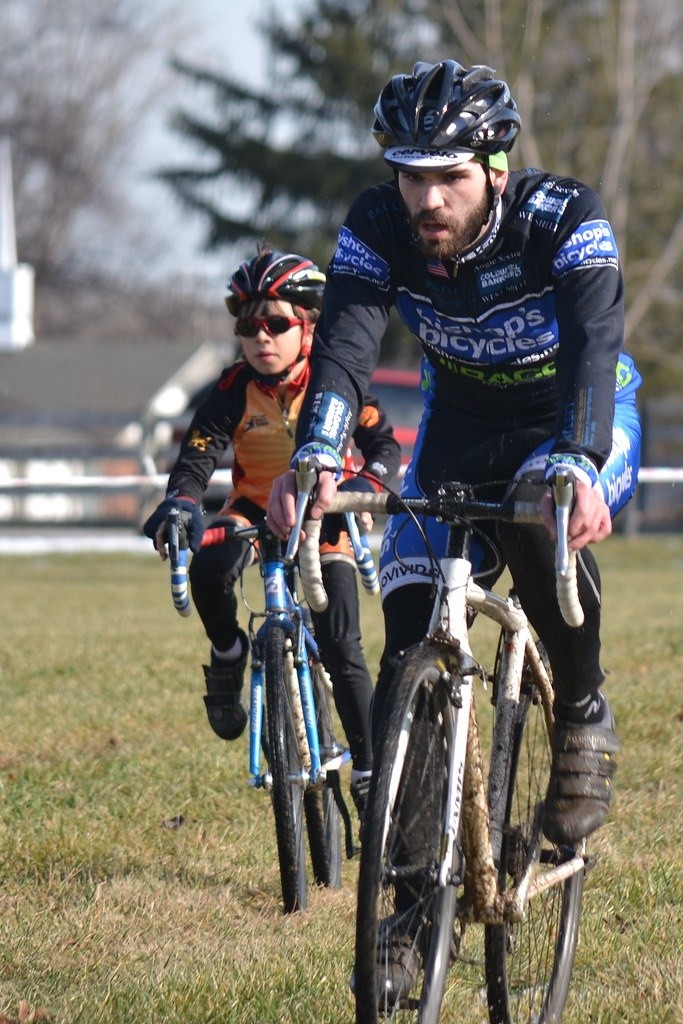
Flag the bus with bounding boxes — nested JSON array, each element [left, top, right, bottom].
[[349, 369, 424, 485]]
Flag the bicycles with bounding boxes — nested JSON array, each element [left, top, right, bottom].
[[286, 451, 588, 1024], [157, 486, 381, 915]]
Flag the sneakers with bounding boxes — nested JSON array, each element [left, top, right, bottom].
[[202, 623, 249, 741], [537, 686, 619, 845], [349, 775, 371, 820], [349, 911, 425, 1001]]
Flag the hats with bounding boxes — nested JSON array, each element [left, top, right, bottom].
[[382, 146, 507, 175]]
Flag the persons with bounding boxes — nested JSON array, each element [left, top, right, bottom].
[[264, 60, 643, 849], [141, 250, 401, 821]]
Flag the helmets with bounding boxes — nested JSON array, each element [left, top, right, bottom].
[[225, 250, 327, 317], [371, 60, 521, 155]]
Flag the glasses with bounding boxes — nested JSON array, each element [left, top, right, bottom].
[[233, 316, 308, 337]]
